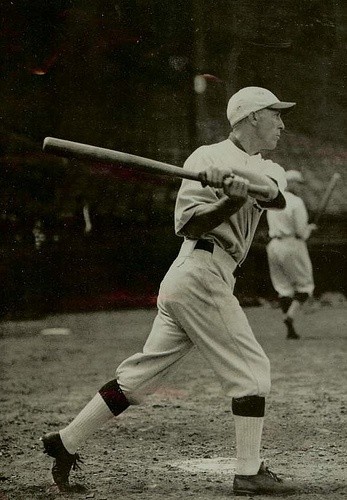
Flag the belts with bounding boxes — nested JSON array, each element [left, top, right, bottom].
[[184, 237, 244, 277]]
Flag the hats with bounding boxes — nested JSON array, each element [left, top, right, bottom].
[[226, 86, 295, 126], [284, 170, 307, 182]]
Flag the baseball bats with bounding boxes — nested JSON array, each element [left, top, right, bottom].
[[39, 136, 270, 199], [312, 171, 341, 224]]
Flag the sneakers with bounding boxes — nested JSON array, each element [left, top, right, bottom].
[[232, 461, 298, 495], [43, 432, 83, 493], [283, 317, 298, 338], [287, 333, 301, 339]]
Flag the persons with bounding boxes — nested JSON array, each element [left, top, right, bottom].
[[40, 88, 300, 494], [267, 169, 319, 339]]
